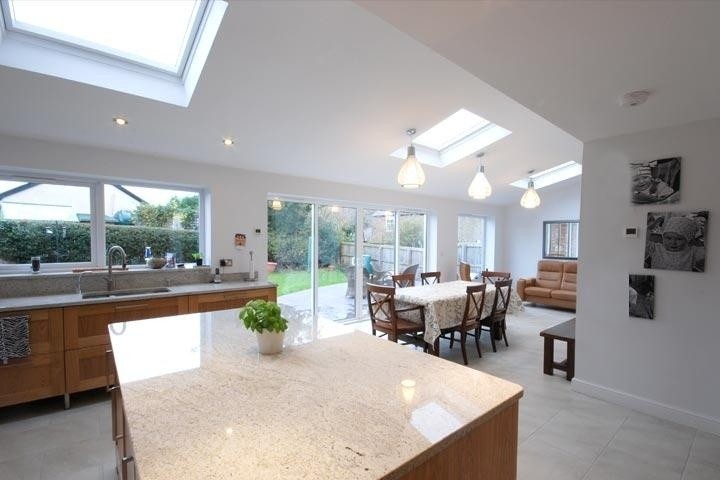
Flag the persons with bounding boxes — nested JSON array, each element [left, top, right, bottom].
[[646, 216, 705, 272], [630, 162, 674, 202]]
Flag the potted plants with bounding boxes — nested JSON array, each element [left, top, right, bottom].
[[238, 297, 289, 356], [146, 233, 175, 269]]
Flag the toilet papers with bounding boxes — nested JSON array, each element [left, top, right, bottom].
[[249, 261, 255, 279]]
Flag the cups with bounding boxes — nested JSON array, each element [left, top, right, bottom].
[[31, 256, 41, 273], [166, 252, 175, 267]]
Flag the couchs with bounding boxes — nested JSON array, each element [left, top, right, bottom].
[[516, 259, 577, 311]]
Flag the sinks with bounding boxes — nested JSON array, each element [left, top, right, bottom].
[[82, 287, 171, 301]]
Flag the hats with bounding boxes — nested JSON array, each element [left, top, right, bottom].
[[662, 217, 697, 242], [632, 164, 651, 176]]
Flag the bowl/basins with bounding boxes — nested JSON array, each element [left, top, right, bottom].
[[147, 259, 166, 269]]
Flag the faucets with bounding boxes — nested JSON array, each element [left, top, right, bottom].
[[108, 246, 127, 276]]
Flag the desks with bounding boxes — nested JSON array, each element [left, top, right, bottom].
[[104, 302, 526, 480], [372, 279, 523, 357], [539, 317, 575, 381]]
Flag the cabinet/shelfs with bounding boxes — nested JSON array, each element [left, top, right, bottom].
[[0, 307, 66, 408], [189, 288, 277, 314], [64, 296, 189, 395]]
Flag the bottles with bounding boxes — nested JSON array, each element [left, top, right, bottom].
[[145, 246, 152, 268]]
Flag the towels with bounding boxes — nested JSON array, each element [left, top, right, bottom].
[[0, 316, 32, 364]]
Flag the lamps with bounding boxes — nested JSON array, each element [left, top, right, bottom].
[[467, 151, 493, 201], [519, 169, 541, 209], [397, 126, 427, 191]]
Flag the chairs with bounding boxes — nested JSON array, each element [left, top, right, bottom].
[[345, 266, 370, 300], [482, 271, 511, 330], [440, 283, 488, 366], [479, 279, 513, 353], [421, 271, 441, 285], [460, 261, 472, 281], [403, 264, 420, 274], [366, 281, 429, 353], [391, 274, 419, 348]]
